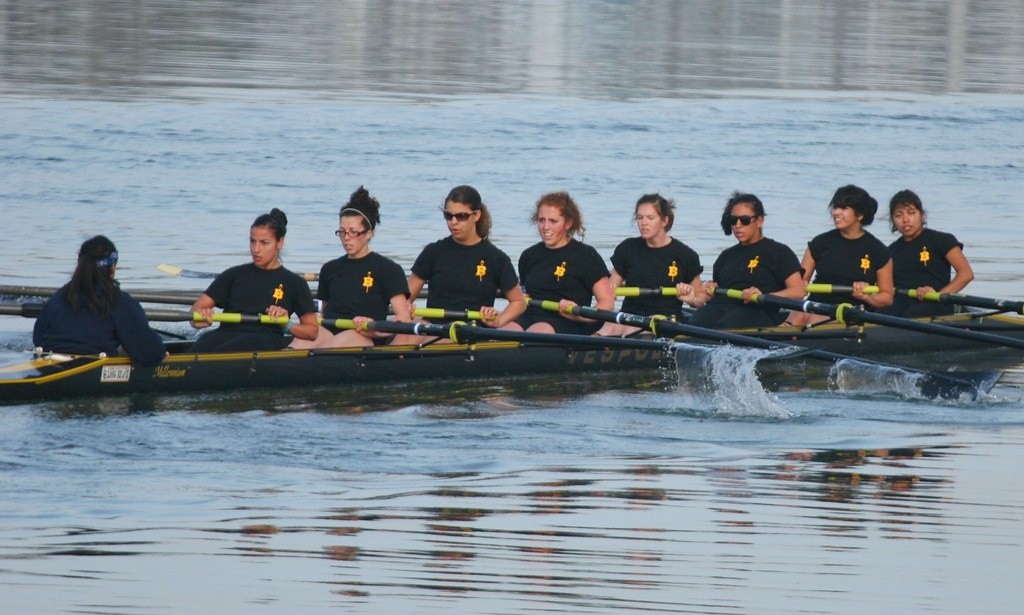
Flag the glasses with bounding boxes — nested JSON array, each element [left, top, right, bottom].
[[727, 215, 757, 226], [442, 209, 476, 222], [335, 230, 368, 238]]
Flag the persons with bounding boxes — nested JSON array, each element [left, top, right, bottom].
[[782, 184, 895, 329], [599, 192, 707, 336], [186, 208, 319, 359], [32, 234, 172, 373], [491, 190, 616, 346], [390, 185, 528, 347], [862, 189, 975, 319], [688, 190, 804, 333], [286, 184, 412, 352]]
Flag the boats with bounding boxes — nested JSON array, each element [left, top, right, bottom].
[[0, 303, 1017, 400]]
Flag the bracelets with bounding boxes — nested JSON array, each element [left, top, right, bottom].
[[281, 321, 293, 332]]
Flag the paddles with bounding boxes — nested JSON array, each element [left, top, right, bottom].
[[1, 265, 1024, 401]]
